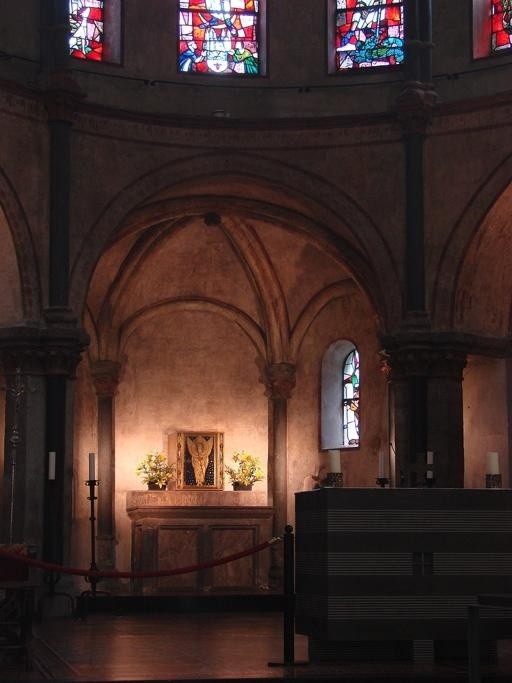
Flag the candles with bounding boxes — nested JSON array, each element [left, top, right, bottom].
[[328, 449, 341, 473], [378, 451, 385, 478], [48, 451, 57, 480], [486, 451, 500, 474], [426, 450, 434, 478], [88, 452, 96, 479]]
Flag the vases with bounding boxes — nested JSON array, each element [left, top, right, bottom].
[[145, 482, 168, 492], [232, 481, 254, 491]]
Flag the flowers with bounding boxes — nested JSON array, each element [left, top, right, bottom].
[[225, 449, 266, 487], [135, 450, 178, 489]]
